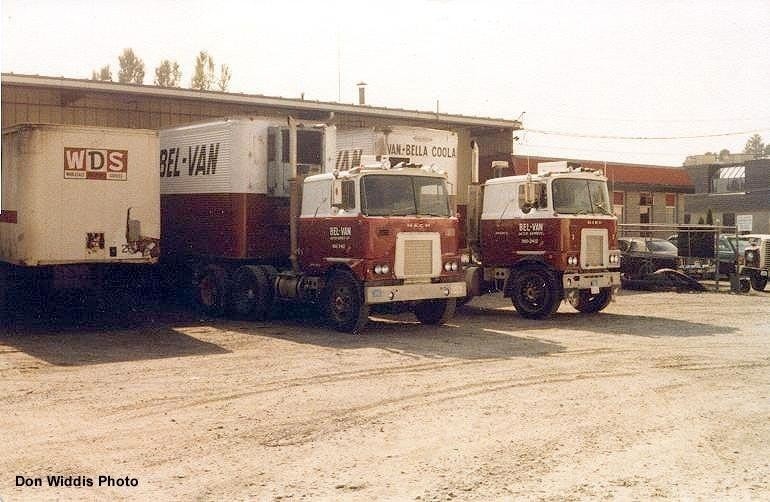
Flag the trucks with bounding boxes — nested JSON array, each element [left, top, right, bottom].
[[0, 122, 160, 325], [161, 114, 470, 338], [336, 126, 620, 318]]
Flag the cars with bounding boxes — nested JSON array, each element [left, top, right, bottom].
[[617, 231, 769, 291]]
